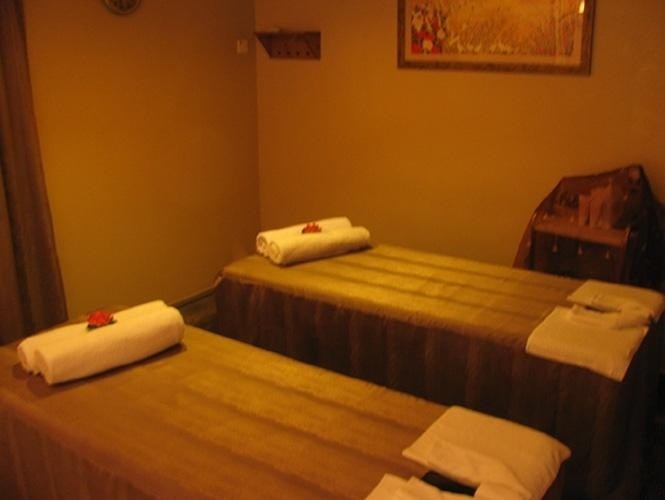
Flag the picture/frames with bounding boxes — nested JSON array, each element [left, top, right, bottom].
[[397, 0, 594, 77]]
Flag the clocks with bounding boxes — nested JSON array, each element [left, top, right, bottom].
[[104, 1, 140, 15]]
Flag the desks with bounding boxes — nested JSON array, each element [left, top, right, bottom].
[[515, 163, 645, 283]]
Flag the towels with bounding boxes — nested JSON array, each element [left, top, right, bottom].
[[18, 299, 187, 387], [257, 216, 375, 266]]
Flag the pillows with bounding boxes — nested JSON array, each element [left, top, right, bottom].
[[524, 278, 662, 384], [365, 401, 570, 499]]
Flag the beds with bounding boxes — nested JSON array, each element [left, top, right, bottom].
[[217, 234, 662, 445], [1, 305, 570, 497]]
